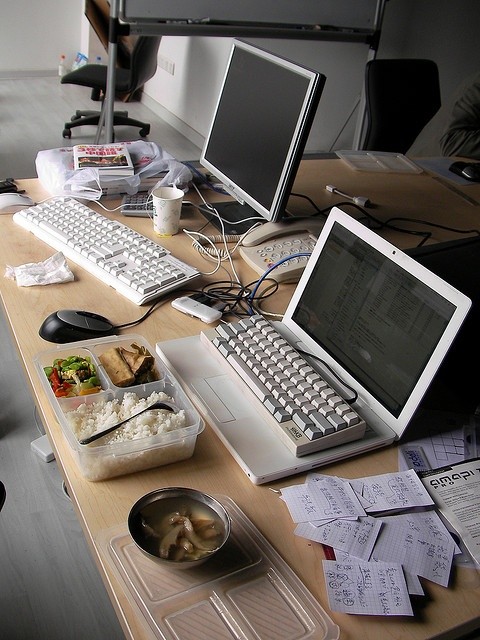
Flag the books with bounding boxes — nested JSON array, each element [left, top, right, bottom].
[[99, 172, 172, 196], [73, 144, 134, 176]]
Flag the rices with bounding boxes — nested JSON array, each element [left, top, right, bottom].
[[63, 390, 188, 445]]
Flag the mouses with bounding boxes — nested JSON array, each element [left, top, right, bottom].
[[449, 161, 478, 181], [1, 193, 36, 214], [39, 310, 114, 343]]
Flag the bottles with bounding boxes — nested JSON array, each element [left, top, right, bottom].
[[97, 57, 101, 65], [58, 55, 66, 82]]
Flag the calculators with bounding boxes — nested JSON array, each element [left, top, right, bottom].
[[120, 192, 154, 218]]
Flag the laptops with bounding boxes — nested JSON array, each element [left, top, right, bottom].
[[154, 207, 472, 486]]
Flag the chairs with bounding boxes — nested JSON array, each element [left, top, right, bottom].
[[363, 58, 443, 154], [59, 34, 163, 138]]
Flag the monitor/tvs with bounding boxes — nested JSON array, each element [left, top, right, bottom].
[[198, 39, 327, 235]]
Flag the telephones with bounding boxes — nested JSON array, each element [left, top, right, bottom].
[[191, 216, 326, 284]]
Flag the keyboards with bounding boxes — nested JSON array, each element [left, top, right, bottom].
[[12, 195, 202, 306], [200, 314, 367, 459]]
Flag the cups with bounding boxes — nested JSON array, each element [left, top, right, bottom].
[[151, 187, 184, 235]]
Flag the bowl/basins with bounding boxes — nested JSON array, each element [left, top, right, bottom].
[[127, 486, 231, 570]]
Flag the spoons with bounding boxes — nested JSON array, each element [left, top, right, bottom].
[[79, 401, 181, 445]]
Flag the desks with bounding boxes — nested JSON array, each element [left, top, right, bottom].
[[0, 153, 479, 640]]
